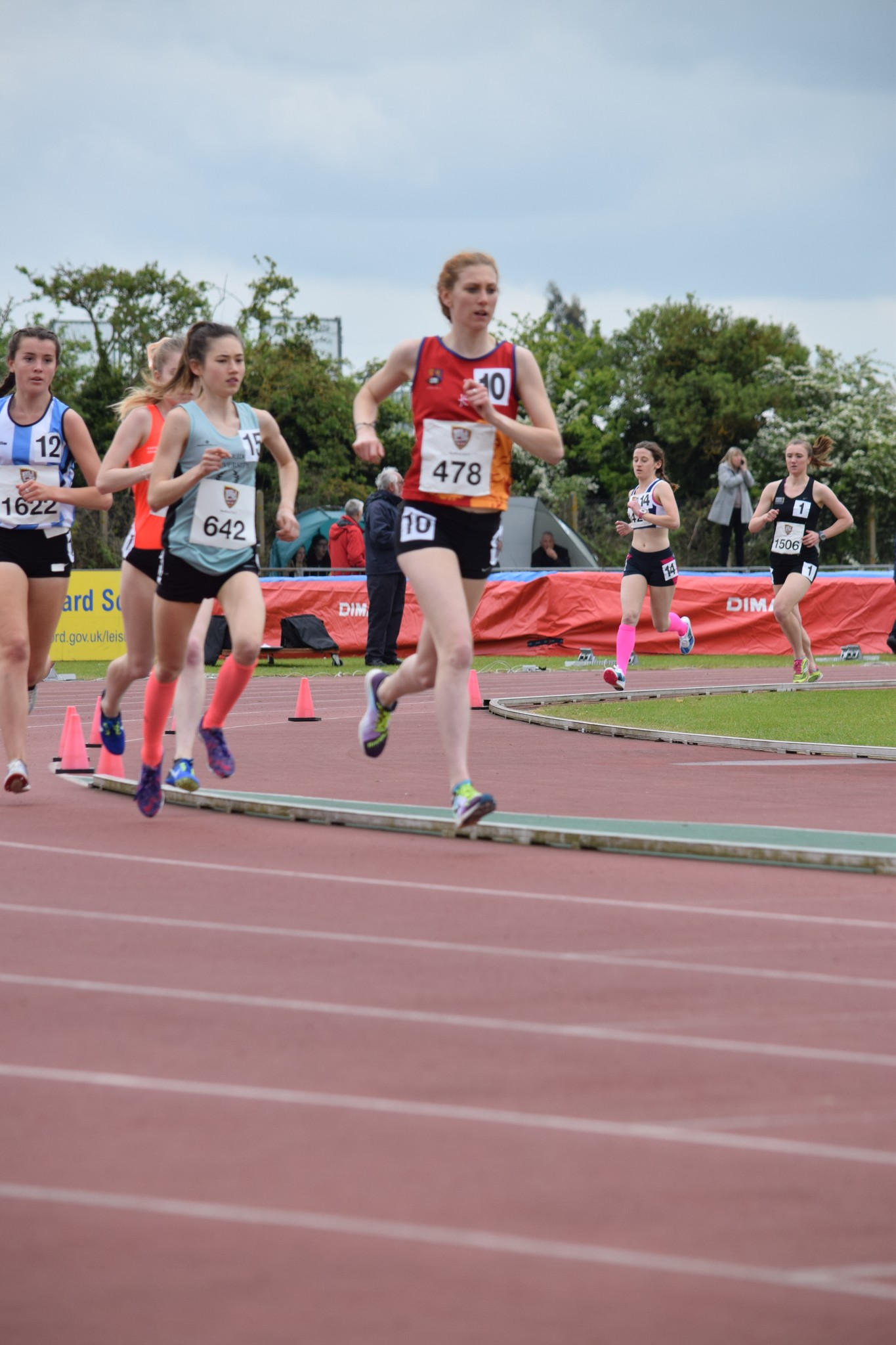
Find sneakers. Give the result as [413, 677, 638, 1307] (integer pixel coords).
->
[806, 665, 823, 683]
[135, 750, 165, 818]
[100, 688, 125, 755]
[677, 616, 694, 655]
[604, 664, 625, 691]
[791, 657, 810, 682]
[359, 668, 398, 758]
[197, 714, 236, 778]
[164, 758, 200, 792]
[452, 784, 496, 830]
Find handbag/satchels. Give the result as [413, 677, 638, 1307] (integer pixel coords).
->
[204, 615, 231, 665]
[280, 613, 338, 650]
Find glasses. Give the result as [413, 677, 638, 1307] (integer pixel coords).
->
[399, 480, 404, 484]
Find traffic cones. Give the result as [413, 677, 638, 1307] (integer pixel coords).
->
[287, 677, 322, 722]
[164, 713, 178, 734]
[467, 669, 489, 711]
[51, 706, 78, 762]
[82, 696, 105, 748]
[55, 714, 100, 774]
[94, 744, 126, 779]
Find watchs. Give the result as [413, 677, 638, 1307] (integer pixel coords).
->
[818, 530, 827, 541]
[639, 507, 646, 519]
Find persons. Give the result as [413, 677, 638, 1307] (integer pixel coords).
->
[491, 518, 504, 568]
[363, 466, 408, 666]
[603, 440, 695, 692]
[747, 433, 854, 683]
[329, 499, 367, 577]
[94, 335, 216, 791]
[0, 326, 114, 793]
[136, 320, 301, 818]
[708, 447, 756, 574]
[285, 545, 310, 577]
[305, 534, 332, 576]
[354, 249, 563, 830]
[531, 531, 571, 568]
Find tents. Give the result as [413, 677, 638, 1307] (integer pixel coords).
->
[268, 504, 365, 577]
[498, 495, 599, 568]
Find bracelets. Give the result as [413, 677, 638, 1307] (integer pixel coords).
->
[354, 420, 375, 426]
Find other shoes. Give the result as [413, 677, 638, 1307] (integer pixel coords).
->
[4, 758, 31, 793]
[365, 661, 384, 666]
[887, 637, 896, 654]
[386, 658, 402, 664]
[28, 685, 37, 713]
